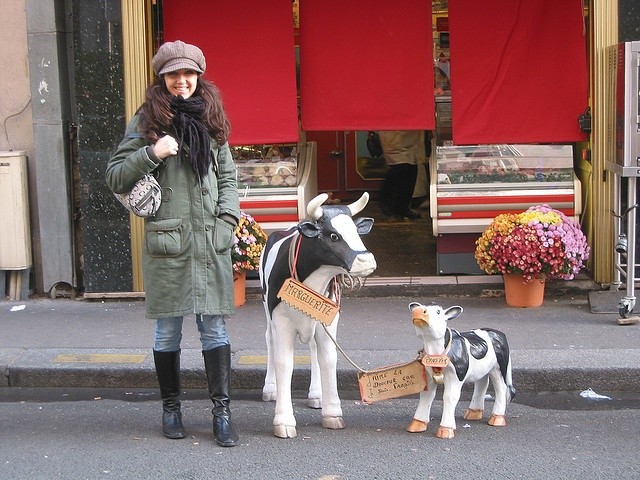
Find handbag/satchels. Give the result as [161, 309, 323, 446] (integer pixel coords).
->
[112, 174, 162, 219]
[366, 133, 382, 160]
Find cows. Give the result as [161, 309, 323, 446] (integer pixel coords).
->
[407, 301, 517, 438]
[259, 192, 378, 439]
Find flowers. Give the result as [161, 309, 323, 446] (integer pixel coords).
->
[475, 203, 590, 283]
[228, 210, 268, 281]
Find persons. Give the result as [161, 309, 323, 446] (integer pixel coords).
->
[376, 130, 422, 223]
[434, 66, 450, 90]
[104, 39, 242, 448]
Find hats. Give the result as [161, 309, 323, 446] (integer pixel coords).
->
[152, 40, 207, 78]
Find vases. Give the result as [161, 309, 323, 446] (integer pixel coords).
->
[234, 272, 246, 307]
[502, 272, 545, 307]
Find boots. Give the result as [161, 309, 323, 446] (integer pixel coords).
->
[202, 344, 240, 447]
[152, 348, 186, 439]
